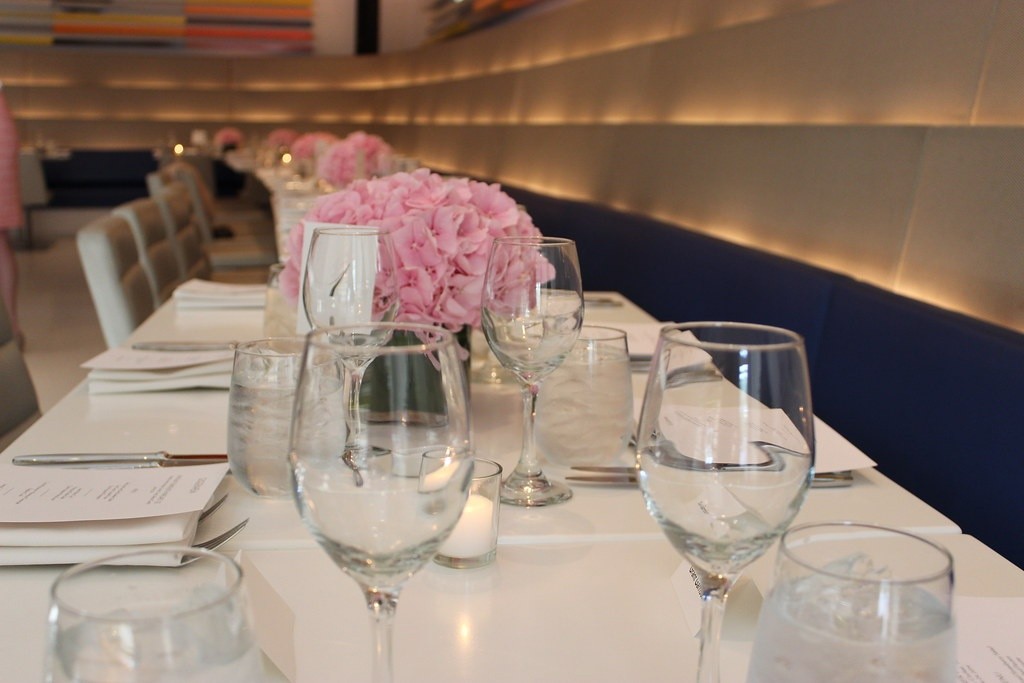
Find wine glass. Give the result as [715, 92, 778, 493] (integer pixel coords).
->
[482, 235, 585, 507]
[285, 327, 469, 683]
[303, 227, 398, 464]
[633, 322, 815, 683]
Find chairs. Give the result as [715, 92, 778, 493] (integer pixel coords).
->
[1, 123, 277, 463]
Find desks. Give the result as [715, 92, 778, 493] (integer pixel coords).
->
[8, 145, 51, 250]
[0, 276, 1024, 683]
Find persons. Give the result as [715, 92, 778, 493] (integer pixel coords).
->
[0, 92, 27, 349]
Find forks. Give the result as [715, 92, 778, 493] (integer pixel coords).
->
[197, 492, 228, 527]
[177, 519, 247, 567]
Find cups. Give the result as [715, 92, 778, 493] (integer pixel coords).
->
[538, 327, 634, 467]
[748, 521, 958, 683]
[228, 338, 307, 500]
[45, 546, 260, 683]
[434, 458, 503, 569]
[262, 264, 307, 336]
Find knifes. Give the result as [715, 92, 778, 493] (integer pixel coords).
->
[564, 466, 852, 488]
[12, 451, 226, 464]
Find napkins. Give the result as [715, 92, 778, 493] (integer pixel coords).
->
[86, 361, 231, 394]
[1, 492, 216, 567]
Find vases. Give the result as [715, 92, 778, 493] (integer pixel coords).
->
[331, 320, 472, 426]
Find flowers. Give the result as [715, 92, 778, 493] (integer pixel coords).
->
[214, 123, 556, 366]
